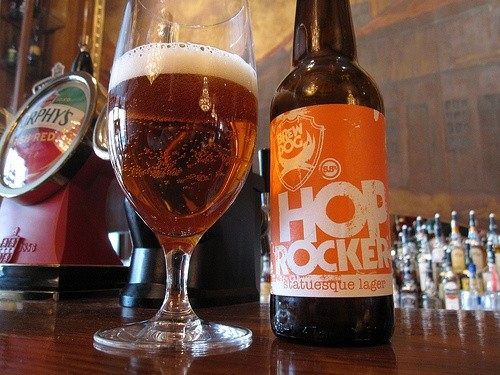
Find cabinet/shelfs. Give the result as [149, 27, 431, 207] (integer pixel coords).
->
[0, 4, 65, 84]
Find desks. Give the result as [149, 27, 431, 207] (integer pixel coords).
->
[0, 294, 500, 375]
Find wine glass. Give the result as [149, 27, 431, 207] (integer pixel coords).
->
[94, 0, 259, 351]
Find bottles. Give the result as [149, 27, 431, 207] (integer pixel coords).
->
[415, 212, 460, 310]
[9, 0, 40, 18]
[71, 35, 93, 76]
[27, 30, 41, 65]
[270, 0, 396, 346]
[392, 218, 419, 308]
[6, 35, 17, 67]
[449, 210, 487, 310]
[258, 192, 270, 303]
[483, 212, 500, 310]
